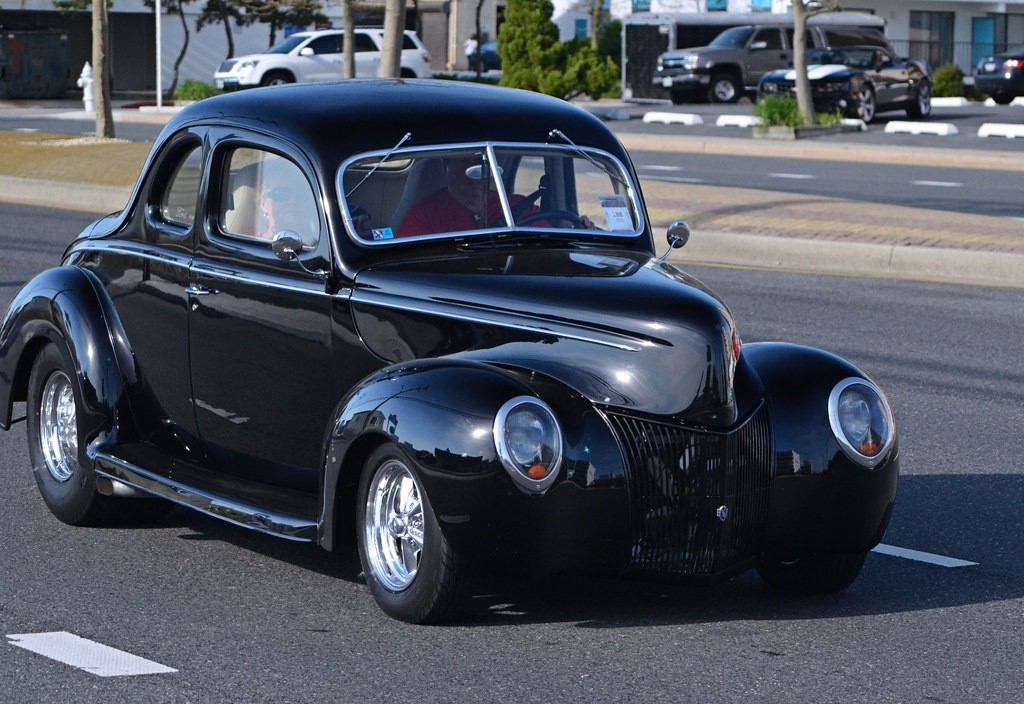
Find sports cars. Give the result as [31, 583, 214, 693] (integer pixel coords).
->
[0, 74, 904, 628]
[755, 44, 935, 124]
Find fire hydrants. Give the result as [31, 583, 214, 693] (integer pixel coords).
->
[76, 61, 95, 112]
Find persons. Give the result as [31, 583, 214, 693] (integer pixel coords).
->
[395, 150, 596, 237]
[463, 33, 479, 71]
[248, 162, 320, 250]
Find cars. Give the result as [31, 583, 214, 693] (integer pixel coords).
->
[469, 39, 502, 74]
[974, 45, 1024, 105]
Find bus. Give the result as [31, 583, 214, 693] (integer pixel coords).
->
[619, 7, 888, 106]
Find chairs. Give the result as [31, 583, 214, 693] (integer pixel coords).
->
[226, 157, 282, 239]
[387, 158, 449, 239]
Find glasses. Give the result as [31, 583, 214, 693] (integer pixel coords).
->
[263, 185, 312, 201]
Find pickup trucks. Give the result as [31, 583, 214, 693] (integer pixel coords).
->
[652, 19, 900, 105]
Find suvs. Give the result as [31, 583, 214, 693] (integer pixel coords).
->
[213, 25, 434, 92]
